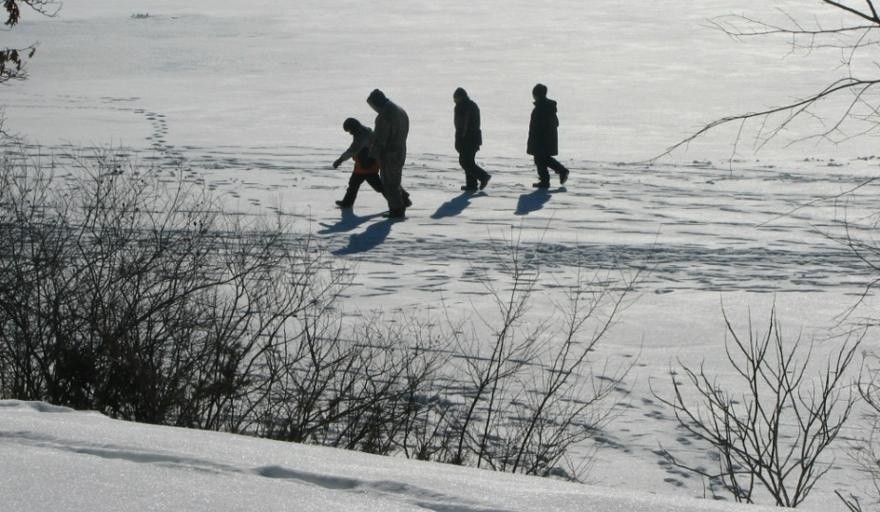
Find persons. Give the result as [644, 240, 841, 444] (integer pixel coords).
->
[333, 118, 386, 206]
[526, 84, 569, 188]
[367, 88, 413, 218]
[453, 88, 491, 190]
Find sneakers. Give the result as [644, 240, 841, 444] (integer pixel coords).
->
[383, 191, 412, 218]
[461, 184, 478, 190]
[336, 200, 353, 208]
[479, 174, 491, 190]
[560, 168, 569, 184]
[533, 181, 549, 188]
